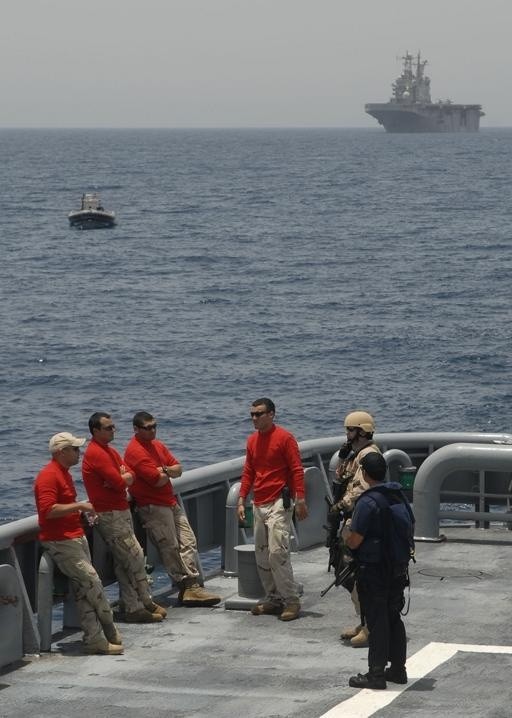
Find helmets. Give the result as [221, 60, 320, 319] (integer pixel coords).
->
[345, 411, 377, 439]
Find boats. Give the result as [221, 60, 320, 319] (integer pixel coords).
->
[68, 194, 117, 230]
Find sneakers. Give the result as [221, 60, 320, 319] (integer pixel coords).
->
[89, 588, 369, 659]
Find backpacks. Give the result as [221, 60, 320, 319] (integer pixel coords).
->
[363, 488, 416, 568]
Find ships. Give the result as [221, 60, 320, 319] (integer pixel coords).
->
[361, 48, 488, 134]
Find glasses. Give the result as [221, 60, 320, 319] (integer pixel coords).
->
[249, 409, 274, 417]
[104, 423, 158, 432]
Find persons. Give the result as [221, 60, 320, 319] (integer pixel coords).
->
[237, 398, 309, 620]
[342, 451, 416, 690]
[82, 412, 167, 622]
[34, 431, 124, 655]
[125, 412, 221, 606]
[328, 411, 383, 647]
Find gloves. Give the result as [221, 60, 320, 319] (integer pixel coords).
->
[330, 502, 346, 512]
[338, 442, 352, 459]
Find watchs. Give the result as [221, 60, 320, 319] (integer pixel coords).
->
[162, 465, 168, 473]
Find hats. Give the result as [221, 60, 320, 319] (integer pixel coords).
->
[49, 431, 86, 453]
[358, 452, 386, 481]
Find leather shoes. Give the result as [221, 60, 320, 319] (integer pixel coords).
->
[349, 663, 410, 688]
